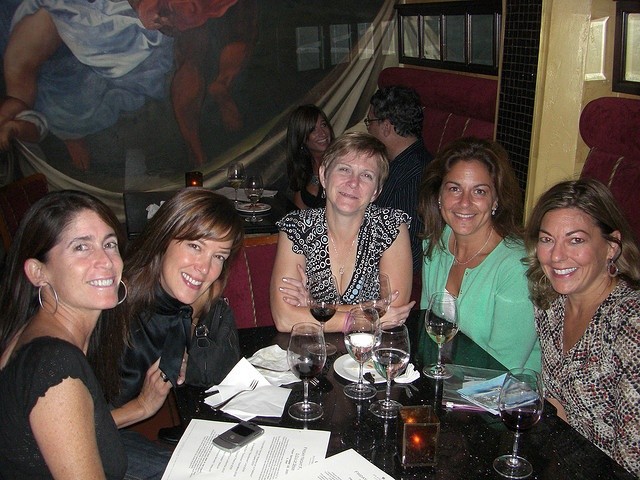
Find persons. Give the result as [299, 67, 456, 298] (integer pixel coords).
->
[108, 187, 244, 441]
[525, 178, 639, 480]
[0, 188, 131, 476]
[358, 82, 440, 269]
[419, 137, 545, 379]
[287, 97, 333, 209]
[270, 130, 415, 338]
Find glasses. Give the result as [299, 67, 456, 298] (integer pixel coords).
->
[364, 117, 384, 126]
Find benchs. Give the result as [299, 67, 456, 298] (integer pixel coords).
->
[220, 234, 281, 330]
[377, 67, 499, 159]
[0, 174, 49, 254]
[574, 98, 640, 250]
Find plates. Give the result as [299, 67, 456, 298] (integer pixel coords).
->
[333, 354, 387, 384]
[235, 203, 271, 214]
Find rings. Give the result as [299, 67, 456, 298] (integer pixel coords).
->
[159, 372, 167, 381]
[296, 302, 302, 307]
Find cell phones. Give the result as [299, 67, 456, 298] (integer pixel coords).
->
[212, 420, 265, 455]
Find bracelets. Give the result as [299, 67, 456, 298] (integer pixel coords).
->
[341, 310, 354, 334]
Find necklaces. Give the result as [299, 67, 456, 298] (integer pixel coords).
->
[309, 172, 320, 185]
[451, 228, 492, 266]
[323, 219, 363, 275]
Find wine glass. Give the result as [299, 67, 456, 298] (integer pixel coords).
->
[369, 320, 411, 419]
[372, 422, 395, 474]
[342, 402, 375, 451]
[358, 273, 391, 332]
[244, 175, 263, 222]
[308, 275, 338, 357]
[422, 292, 460, 379]
[343, 307, 378, 400]
[493, 368, 544, 479]
[287, 322, 326, 422]
[227, 161, 247, 205]
